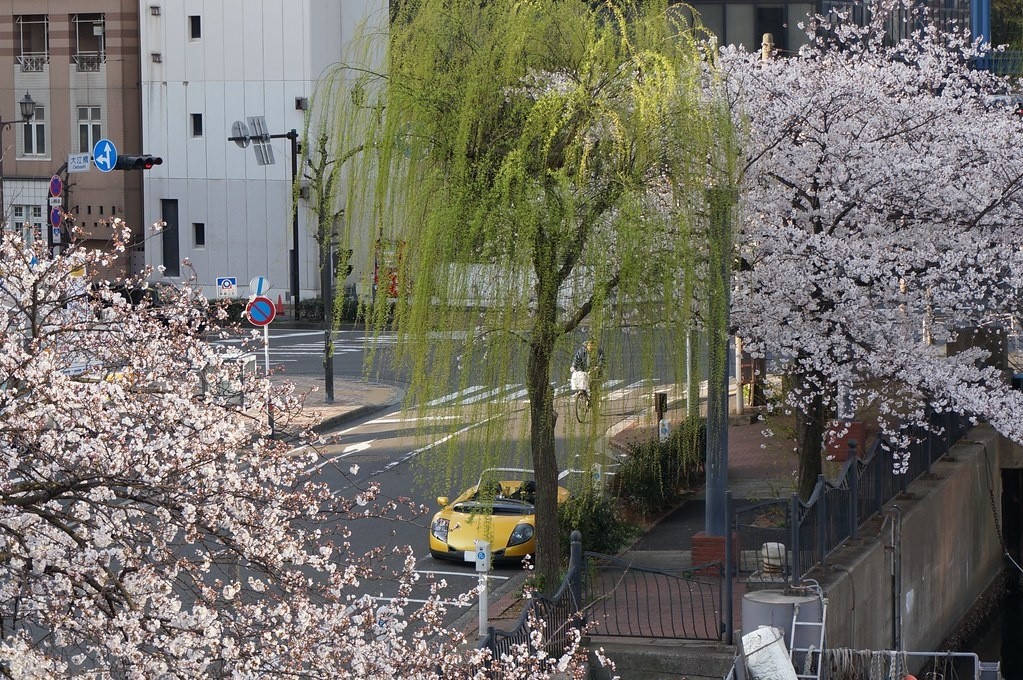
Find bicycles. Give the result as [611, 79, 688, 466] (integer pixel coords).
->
[570, 368, 604, 422]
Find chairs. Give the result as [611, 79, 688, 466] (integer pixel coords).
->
[474, 481, 506, 501]
[509, 481, 535, 505]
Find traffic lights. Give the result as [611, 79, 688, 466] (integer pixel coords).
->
[122, 152, 163, 170]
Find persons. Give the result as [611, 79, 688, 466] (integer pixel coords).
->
[896, 277, 937, 348]
[570, 338, 606, 415]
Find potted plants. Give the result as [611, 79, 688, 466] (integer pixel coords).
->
[559, 418, 707, 573]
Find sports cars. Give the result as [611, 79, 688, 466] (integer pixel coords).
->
[428, 479, 573, 564]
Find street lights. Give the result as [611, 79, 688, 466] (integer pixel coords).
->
[0, 88, 37, 262]
